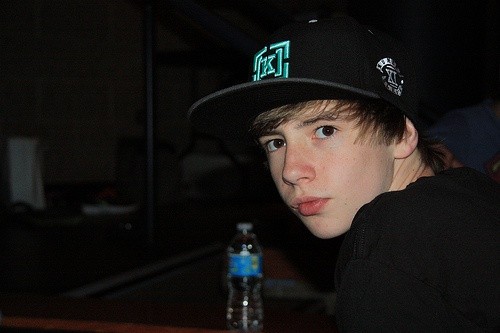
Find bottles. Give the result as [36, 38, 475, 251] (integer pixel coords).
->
[226, 222, 264, 333]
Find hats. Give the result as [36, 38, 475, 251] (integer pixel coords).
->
[188, 15, 418, 137]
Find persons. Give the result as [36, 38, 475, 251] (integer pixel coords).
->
[423, 95, 500, 187]
[187, 17, 500, 333]
